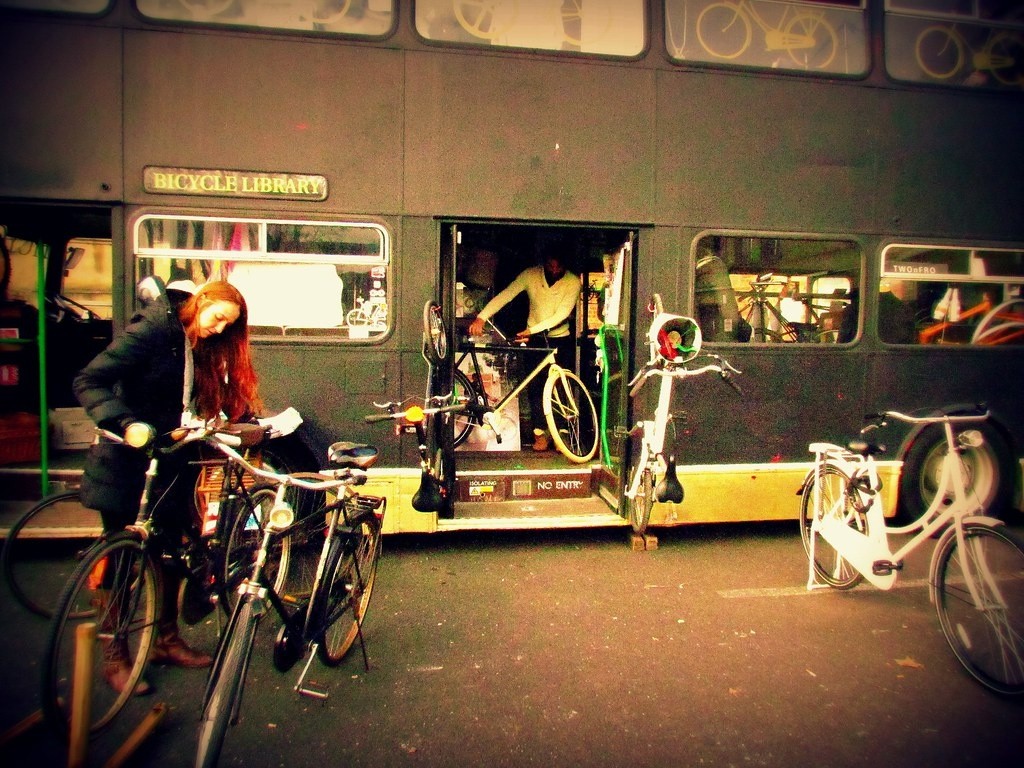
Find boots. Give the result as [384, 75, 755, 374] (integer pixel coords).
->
[147, 560, 213, 668]
[96, 589, 149, 695]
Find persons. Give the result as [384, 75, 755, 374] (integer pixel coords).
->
[72, 281, 262, 696]
[468, 241, 581, 451]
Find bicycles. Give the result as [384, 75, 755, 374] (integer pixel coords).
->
[745, 268, 852, 345]
[0, 406, 297, 739]
[796, 411, 1024, 700]
[446, 322, 601, 464]
[180, 428, 387, 767]
[364, 298, 494, 515]
[623, 294, 746, 539]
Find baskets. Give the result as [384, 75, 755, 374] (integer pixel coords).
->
[650, 313, 702, 363]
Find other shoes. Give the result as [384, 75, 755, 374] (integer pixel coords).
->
[556, 429, 570, 452]
[533, 428, 548, 450]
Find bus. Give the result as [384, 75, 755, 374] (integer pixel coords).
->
[0, 1, 1023, 542]
